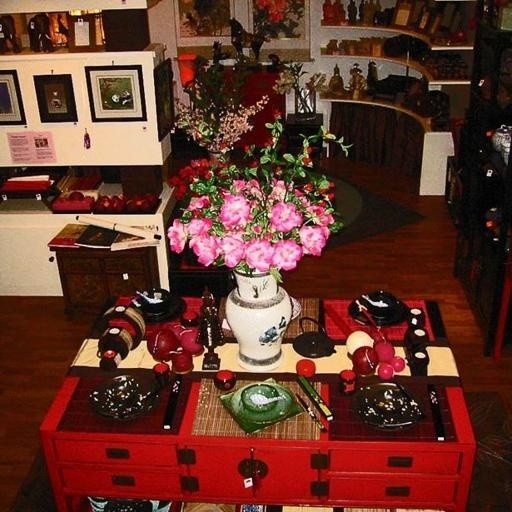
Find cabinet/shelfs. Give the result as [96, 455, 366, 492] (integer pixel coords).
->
[0, 0, 179, 300]
[36, 292, 475, 512]
[318, 18, 477, 197]
[51, 242, 159, 316]
[284, 111, 323, 156]
[454, 0, 512, 360]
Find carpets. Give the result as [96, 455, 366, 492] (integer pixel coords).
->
[281, 145, 430, 257]
[10, 385, 511, 512]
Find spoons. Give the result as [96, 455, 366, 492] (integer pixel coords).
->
[133, 288, 163, 305]
[361, 293, 389, 310]
[249, 393, 286, 405]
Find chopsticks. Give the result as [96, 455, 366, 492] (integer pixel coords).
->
[294, 391, 328, 437]
[393, 374, 422, 418]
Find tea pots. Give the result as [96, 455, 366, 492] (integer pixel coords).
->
[293, 315, 339, 358]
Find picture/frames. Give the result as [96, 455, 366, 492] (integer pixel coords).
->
[0, 68, 28, 127]
[84, 65, 148, 125]
[32, 72, 80, 125]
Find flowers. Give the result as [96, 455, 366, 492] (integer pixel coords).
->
[272, 59, 330, 112]
[160, 117, 355, 283]
[167, 57, 271, 158]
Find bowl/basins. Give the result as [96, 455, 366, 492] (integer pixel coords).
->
[87, 372, 161, 420]
[350, 380, 423, 429]
[360, 288, 401, 321]
[137, 287, 176, 316]
[240, 384, 279, 412]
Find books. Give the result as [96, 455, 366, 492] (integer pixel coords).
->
[110, 224, 161, 252]
[74, 224, 120, 249]
[47, 224, 90, 249]
[67, 171, 104, 192]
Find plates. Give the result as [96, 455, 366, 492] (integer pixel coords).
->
[219, 376, 304, 437]
[229, 381, 295, 424]
[347, 299, 410, 329]
[128, 294, 187, 324]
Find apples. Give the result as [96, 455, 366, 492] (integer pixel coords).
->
[352, 346, 378, 374]
[147, 328, 178, 362]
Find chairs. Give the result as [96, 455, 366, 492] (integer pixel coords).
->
[202, 62, 286, 156]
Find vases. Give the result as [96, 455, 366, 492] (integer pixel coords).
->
[225, 261, 294, 372]
